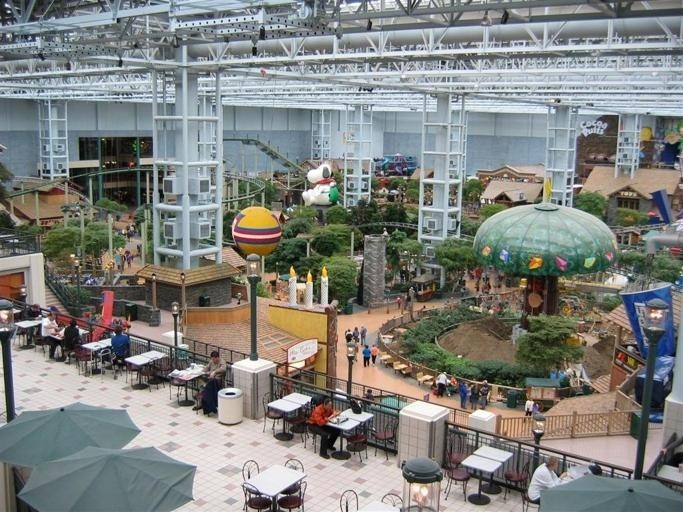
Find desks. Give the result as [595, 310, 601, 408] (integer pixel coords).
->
[472, 446, 513, 495]
[461, 454, 501, 505]
[359, 500, 399, 511]
[241, 464, 308, 512]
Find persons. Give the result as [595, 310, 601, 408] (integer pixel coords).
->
[40, 313, 64, 361]
[197, 350, 226, 386]
[522, 397, 534, 422]
[435, 370, 447, 397]
[307, 397, 339, 460]
[478, 379, 489, 409]
[191, 375, 214, 413]
[111, 325, 130, 367]
[397, 293, 411, 311]
[445, 375, 457, 397]
[527, 455, 567, 507]
[458, 381, 467, 409]
[62, 319, 80, 351]
[459, 271, 505, 298]
[19, 304, 41, 347]
[469, 384, 477, 409]
[344, 323, 378, 367]
[105, 210, 142, 268]
[363, 388, 375, 407]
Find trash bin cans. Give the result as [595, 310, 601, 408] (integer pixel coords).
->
[345, 303, 353, 315]
[125, 303, 137, 321]
[507, 389, 517, 408]
[630, 412, 649, 440]
[217, 387, 244, 425]
[199, 296, 210, 307]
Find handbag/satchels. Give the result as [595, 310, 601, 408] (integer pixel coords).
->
[350, 399, 361, 414]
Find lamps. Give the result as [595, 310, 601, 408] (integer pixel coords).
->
[25, 16, 274, 77]
[498, 7, 511, 25]
[363, 17, 375, 32]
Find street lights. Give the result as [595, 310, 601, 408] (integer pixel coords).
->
[69, 254, 74, 285]
[179, 272, 185, 315]
[399, 457, 443, 512]
[171, 301, 178, 369]
[0, 299, 15, 424]
[633, 297, 668, 480]
[346, 340, 356, 400]
[73, 257, 80, 304]
[18, 284, 26, 304]
[150, 273, 156, 310]
[246, 254, 261, 361]
[531, 414, 546, 478]
[107, 260, 114, 286]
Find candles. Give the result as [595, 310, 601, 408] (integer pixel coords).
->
[287, 266, 298, 303]
[304, 269, 313, 306]
[320, 267, 330, 303]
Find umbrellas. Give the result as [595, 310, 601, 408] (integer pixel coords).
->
[538, 472, 683, 511]
[0, 400, 141, 469]
[11, 441, 197, 512]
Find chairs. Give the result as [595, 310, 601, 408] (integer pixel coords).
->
[339, 489, 359, 511]
[440, 429, 470, 502]
[242, 459, 260, 481]
[503, 459, 540, 511]
[277, 481, 308, 512]
[379, 349, 526, 407]
[374, 418, 399, 460]
[241, 483, 273, 512]
[0, 304, 226, 418]
[284, 458, 305, 474]
[381, 492, 403, 507]
[261, 387, 374, 463]
